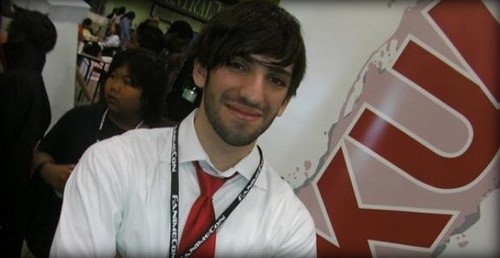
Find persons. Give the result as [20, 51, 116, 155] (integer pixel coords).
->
[79, 3, 205, 119]
[48, 0, 317, 258]
[0, 2, 58, 258]
[20, 48, 166, 258]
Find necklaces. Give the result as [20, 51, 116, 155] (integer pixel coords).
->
[94, 108, 144, 144]
[169, 122, 264, 258]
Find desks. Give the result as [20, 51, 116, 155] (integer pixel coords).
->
[77, 41, 112, 105]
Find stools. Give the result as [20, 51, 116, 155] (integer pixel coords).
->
[85, 67, 107, 92]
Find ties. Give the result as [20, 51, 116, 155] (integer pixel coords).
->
[177, 160, 241, 258]
